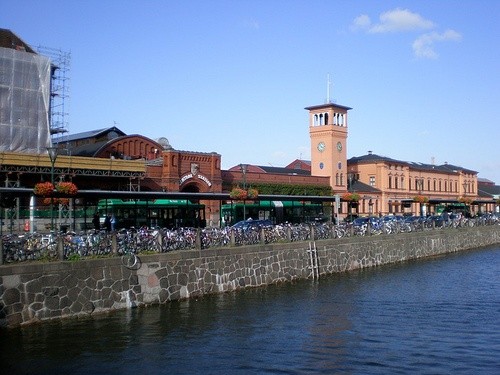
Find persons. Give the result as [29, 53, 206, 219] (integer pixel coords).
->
[93, 212, 125, 232]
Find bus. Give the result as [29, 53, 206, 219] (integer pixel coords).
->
[435, 203, 470, 218]
[222, 199, 325, 224]
[98, 198, 197, 230]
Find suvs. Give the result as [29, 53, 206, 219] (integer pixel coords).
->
[233, 219, 273, 229]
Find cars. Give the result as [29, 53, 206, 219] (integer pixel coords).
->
[350, 216, 380, 226]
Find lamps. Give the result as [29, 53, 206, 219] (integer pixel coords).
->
[317, 142, 325, 152]
[337, 142, 342, 151]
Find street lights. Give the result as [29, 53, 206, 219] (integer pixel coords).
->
[46, 146, 61, 232]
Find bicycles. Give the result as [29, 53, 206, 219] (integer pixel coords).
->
[236, 221, 377, 246]
[376, 210, 500, 234]
[0, 225, 237, 264]
[122, 235, 139, 269]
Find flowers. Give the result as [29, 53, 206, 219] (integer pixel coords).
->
[459, 198, 473, 203]
[230, 188, 258, 200]
[342, 193, 361, 201]
[413, 196, 429, 203]
[34, 181, 77, 197]
